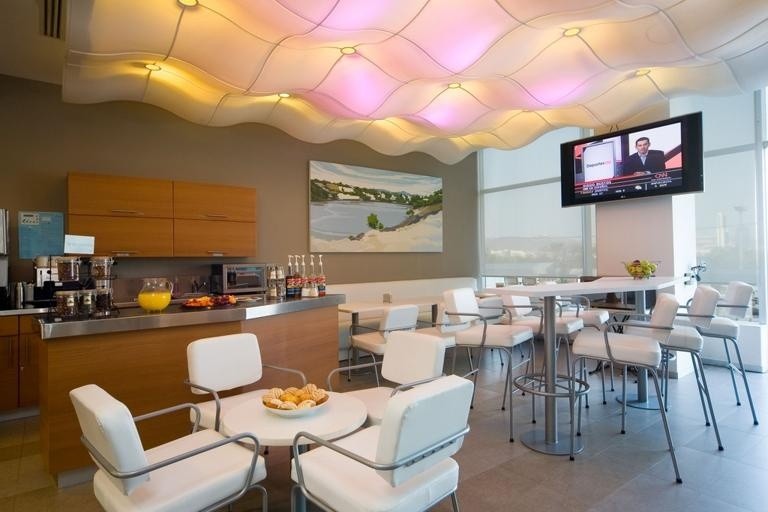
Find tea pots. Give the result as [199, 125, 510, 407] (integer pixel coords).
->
[137, 278, 173, 312]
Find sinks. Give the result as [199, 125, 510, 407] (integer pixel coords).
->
[184, 293, 209, 296]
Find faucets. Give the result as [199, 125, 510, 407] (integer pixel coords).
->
[193, 280, 207, 292]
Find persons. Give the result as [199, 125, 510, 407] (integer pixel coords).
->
[623, 137, 665, 176]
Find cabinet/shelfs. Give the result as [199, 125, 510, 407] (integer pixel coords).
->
[64, 172, 258, 257]
[1, 314, 39, 412]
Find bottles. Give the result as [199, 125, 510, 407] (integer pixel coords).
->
[265, 264, 287, 296]
[10, 282, 35, 309]
[173, 279, 180, 299]
[55, 256, 113, 321]
[302, 283, 311, 297]
[310, 283, 319, 297]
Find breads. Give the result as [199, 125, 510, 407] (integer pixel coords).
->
[262, 383, 329, 410]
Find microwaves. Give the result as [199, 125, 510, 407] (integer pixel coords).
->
[209, 263, 267, 294]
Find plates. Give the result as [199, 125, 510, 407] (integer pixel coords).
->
[260, 394, 331, 418]
[181, 303, 211, 309]
[235, 297, 263, 302]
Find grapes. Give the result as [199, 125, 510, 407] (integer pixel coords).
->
[210, 295, 228, 305]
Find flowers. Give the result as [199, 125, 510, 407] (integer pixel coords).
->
[622, 260, 658, 277]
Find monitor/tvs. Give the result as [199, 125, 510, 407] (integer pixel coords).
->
[560, 111, 704, 206]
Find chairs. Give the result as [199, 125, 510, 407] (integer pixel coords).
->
[569, 293, 682, 483]
[290, 374, 474, 512]
[664, 280, 760, 425]
[622, 286, 724, 450]
[187, 333, 307, 444]
[70, 383, 268, 511]
